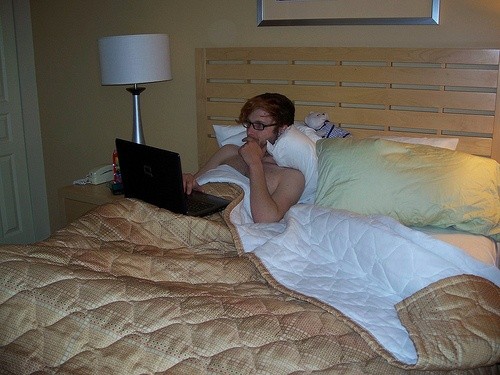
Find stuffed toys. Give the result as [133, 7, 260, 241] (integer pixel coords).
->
[304, 110, 353, 139]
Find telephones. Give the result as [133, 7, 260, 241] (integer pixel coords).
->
[72, 164, 114, 185]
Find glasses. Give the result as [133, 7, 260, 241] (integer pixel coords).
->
[241, 120, 279, 130]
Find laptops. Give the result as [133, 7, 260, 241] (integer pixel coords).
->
[115, 137, 232, 217]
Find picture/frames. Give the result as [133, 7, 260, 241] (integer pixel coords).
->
[255, 0, 439, 26]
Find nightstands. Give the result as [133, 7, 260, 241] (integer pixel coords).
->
[65, 180, 124, 221]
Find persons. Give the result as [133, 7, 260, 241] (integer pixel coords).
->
[182, 92, 306, 223]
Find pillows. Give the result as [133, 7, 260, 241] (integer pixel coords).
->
[212, 123, 500, 242]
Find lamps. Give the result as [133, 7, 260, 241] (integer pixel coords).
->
[97, 34, 173, 145]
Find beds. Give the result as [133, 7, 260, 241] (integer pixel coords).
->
[0, 47, 500, 375]
